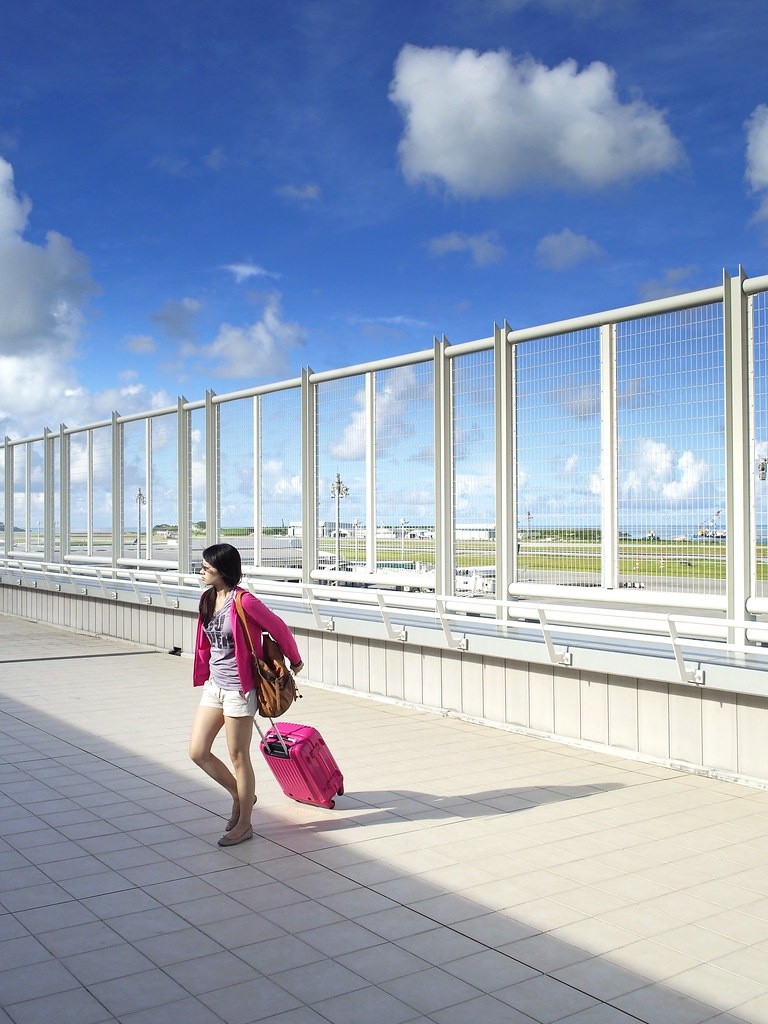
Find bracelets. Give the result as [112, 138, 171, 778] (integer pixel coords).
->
[290, 660, 302, 667]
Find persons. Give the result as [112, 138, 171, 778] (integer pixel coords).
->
[188, 543, 304, 847]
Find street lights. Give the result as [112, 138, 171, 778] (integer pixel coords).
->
[135, 487, 147, 571]
[351, 518, 363, 562]
[328, 473, 350, 587]
[399, 517, 410, 561]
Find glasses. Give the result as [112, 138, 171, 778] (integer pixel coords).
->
[199, 561, 220, 576]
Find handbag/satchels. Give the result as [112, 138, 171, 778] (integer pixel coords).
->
[235, 590, 303, 718]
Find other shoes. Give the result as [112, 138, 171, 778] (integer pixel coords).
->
[217, 823, 253, 847]
[224, 795, 258, 832]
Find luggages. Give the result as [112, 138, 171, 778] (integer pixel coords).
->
[252, 716, 344, 809]
